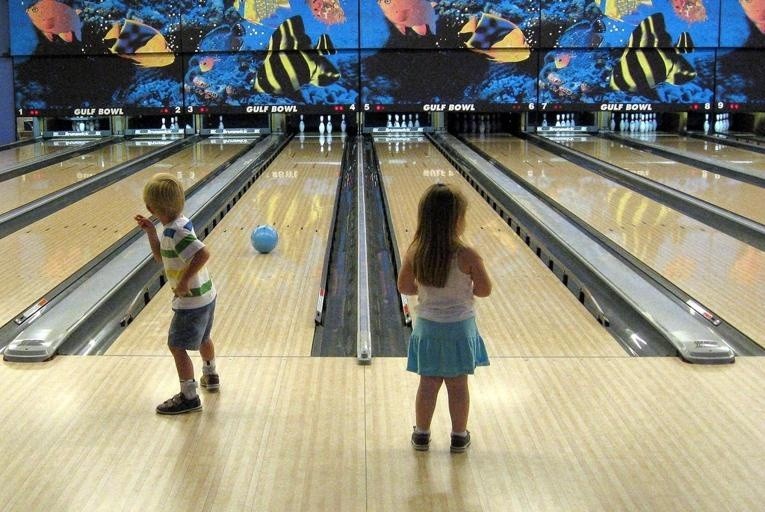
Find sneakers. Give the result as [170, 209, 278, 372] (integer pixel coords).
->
[411, 425, 432, 452]
[200, 373, 220, 391]
[155, 391, 203, 414]
[449, 430, 472, 452]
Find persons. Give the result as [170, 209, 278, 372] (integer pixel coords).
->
[134, 172, 221, 417]
[394, 182, 494, 455]
